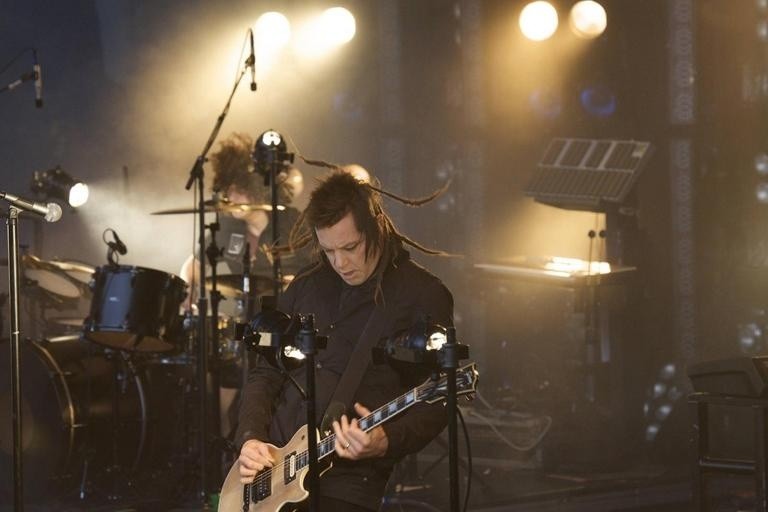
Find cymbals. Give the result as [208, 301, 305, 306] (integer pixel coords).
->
[205, 273, 288, 297]
[148, 202, 289, 215]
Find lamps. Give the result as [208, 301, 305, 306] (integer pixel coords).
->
[24, 165, 91, 210]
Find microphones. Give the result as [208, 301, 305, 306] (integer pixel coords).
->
[31, 47, 45, 111]
[248, 29, 259, 94]
[0, 189, 64, 225]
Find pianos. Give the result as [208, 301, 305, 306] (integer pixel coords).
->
[522, 138, 655, 204]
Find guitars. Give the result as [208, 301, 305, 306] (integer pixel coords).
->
[215, 360, 478, 511]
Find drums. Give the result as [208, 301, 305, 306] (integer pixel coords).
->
[46, 261, 95, 290]
[47, 316, 88, 339]
[21, 269, 80, 312]
[165, 308, 235, 357]
[1, 333, 154, 512]
[80, 263, 189, 357]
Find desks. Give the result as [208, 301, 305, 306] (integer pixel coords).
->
[688, 392, 768, 511]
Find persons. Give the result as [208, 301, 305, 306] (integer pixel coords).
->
[174, 133, 317, 448]
[229, 169, 460, 511]
